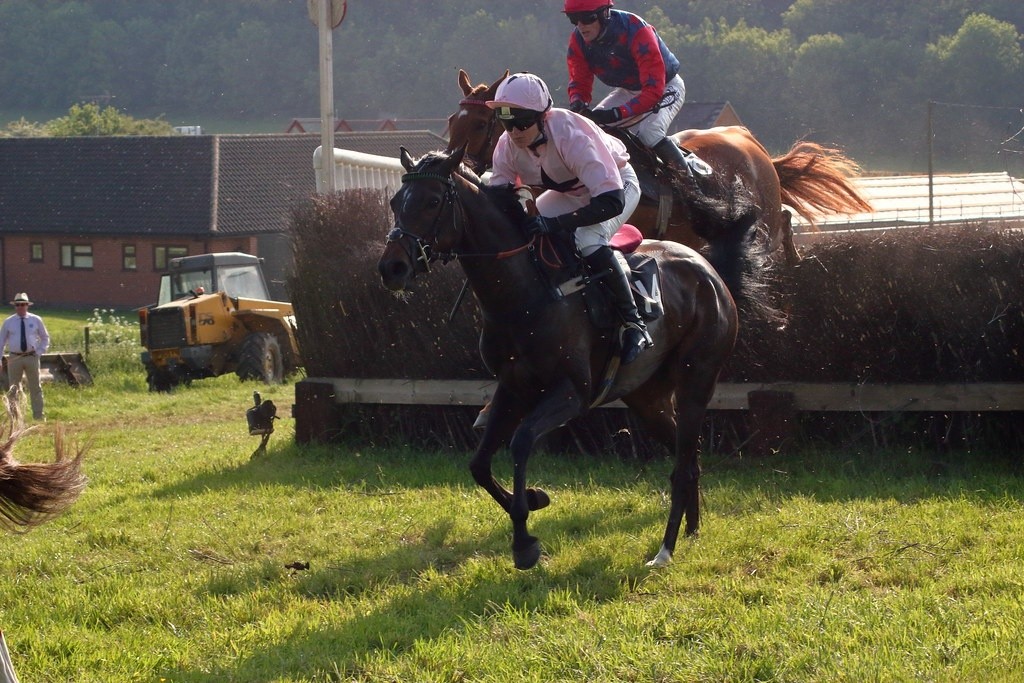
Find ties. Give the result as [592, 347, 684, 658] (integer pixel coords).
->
[20, 317, 27, 352]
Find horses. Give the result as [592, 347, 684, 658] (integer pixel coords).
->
[446, 70, 873, 460]
[378, 146, 788, 571]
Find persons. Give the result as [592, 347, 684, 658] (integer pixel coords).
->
[480, 70, 654, 365]
[0, 292, 50, 422]
[561, 0, 703, 196]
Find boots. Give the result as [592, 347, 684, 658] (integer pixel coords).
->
[652, 137, 703, 206]
[582, 245, 654, 364]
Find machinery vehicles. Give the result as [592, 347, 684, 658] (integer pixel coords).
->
[139, 250, 303, 395]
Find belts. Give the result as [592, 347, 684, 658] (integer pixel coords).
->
[11, 352, 34, 356]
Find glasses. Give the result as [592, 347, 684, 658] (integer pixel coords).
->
[14, 303, 25, 306]
[500, 119, 537, 132]
[570, 12, 599, 25]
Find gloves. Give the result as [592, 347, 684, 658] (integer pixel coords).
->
[522, 215, 561, 236]
[569, 100, 622, 127]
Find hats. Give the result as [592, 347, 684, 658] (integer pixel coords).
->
[485, 72, 554, 112]
[10, 293, 34, 305]
[560, 0, 614, 13]
